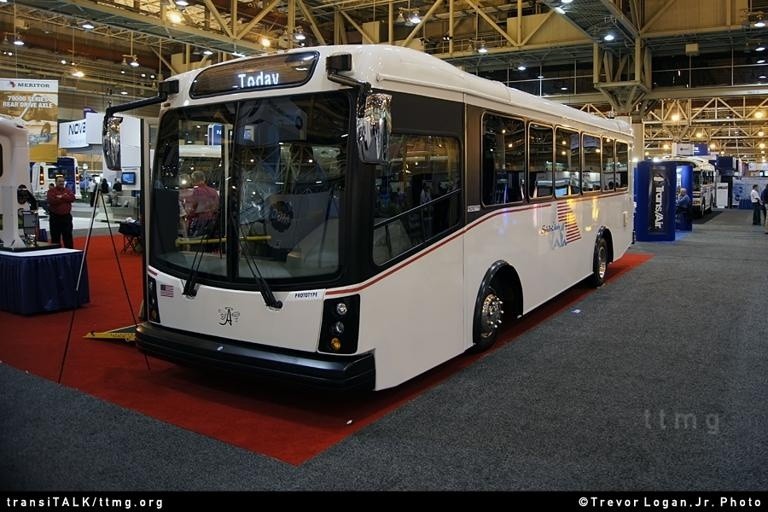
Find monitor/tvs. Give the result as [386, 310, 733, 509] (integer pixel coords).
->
[23, 213, 39, 228]
[121, 172, 135, 184]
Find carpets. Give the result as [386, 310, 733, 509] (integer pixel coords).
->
[0, 233, 653, 466]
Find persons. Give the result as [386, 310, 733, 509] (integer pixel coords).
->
[675, 188, 690, 214]
[289, 140, 328, 193]
[186, 170, 220, 254]
[49, 183, 54, 189]
[47, 176, 75, 249]
[90, 178, 122, 207]
[751, 184, 762, 225]
[238, 138, 280, 256]
[761, 184, 768, 226]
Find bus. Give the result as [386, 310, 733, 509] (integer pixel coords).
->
[102, 44, 634, 395]
[653, 155, 717, 217]
[30, 155, 82, 213]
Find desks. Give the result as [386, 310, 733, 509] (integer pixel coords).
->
[118, 221, 141, 255]
[0, 240, 90, 316]
